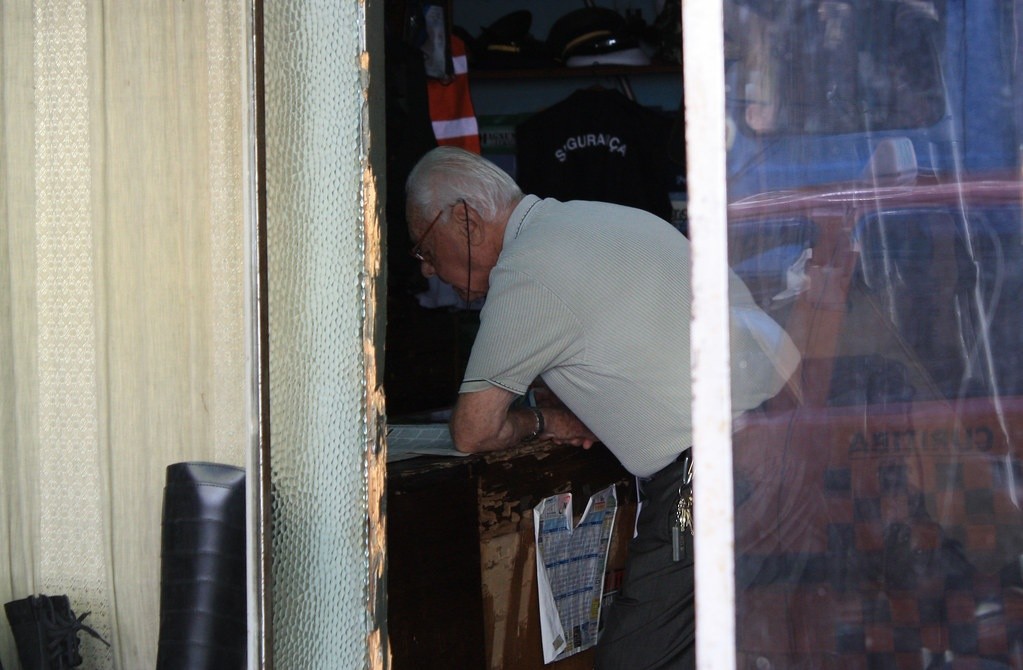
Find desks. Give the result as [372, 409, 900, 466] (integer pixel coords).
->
[388, 404, 642, 670]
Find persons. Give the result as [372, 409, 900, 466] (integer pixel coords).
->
[405, 146, 828, 668]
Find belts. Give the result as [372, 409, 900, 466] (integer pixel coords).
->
[671, 397, 793, 464]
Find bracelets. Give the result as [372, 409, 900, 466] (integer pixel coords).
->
[522, 401, 546, 441]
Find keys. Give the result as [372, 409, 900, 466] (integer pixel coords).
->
[674, 486, 695, 534]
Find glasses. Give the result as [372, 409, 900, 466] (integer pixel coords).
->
[409, 210, 443, 261]
